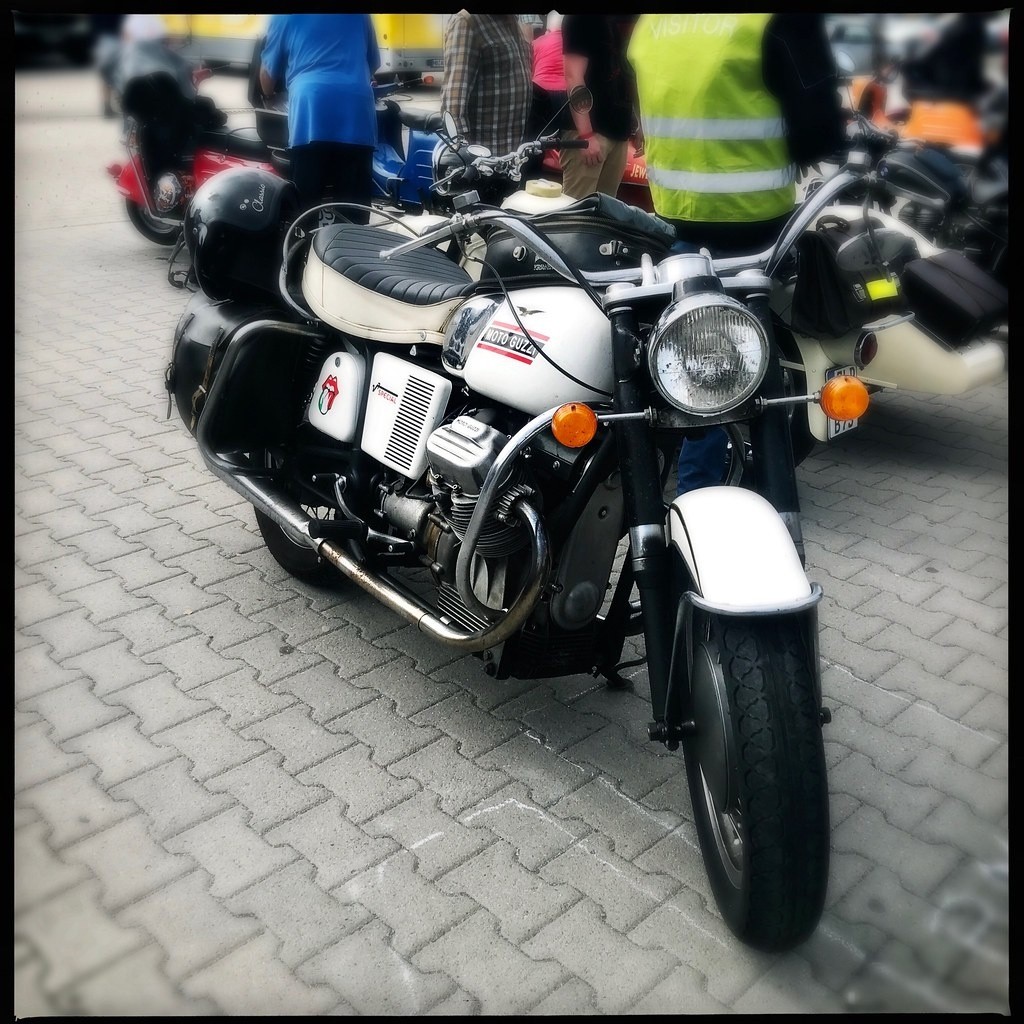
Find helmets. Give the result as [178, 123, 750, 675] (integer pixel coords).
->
[183, 167, 303, 301]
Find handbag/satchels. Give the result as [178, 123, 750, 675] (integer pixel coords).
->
[164, 292, 301, 442]
[793, 214, 887, 342]
[479, 195, 677, 283]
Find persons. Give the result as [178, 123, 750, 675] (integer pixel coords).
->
[623, 8, 852, 512]
[85, 6, 644, 210]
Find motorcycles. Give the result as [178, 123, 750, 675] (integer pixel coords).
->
[91, 8, 1013, 951]
